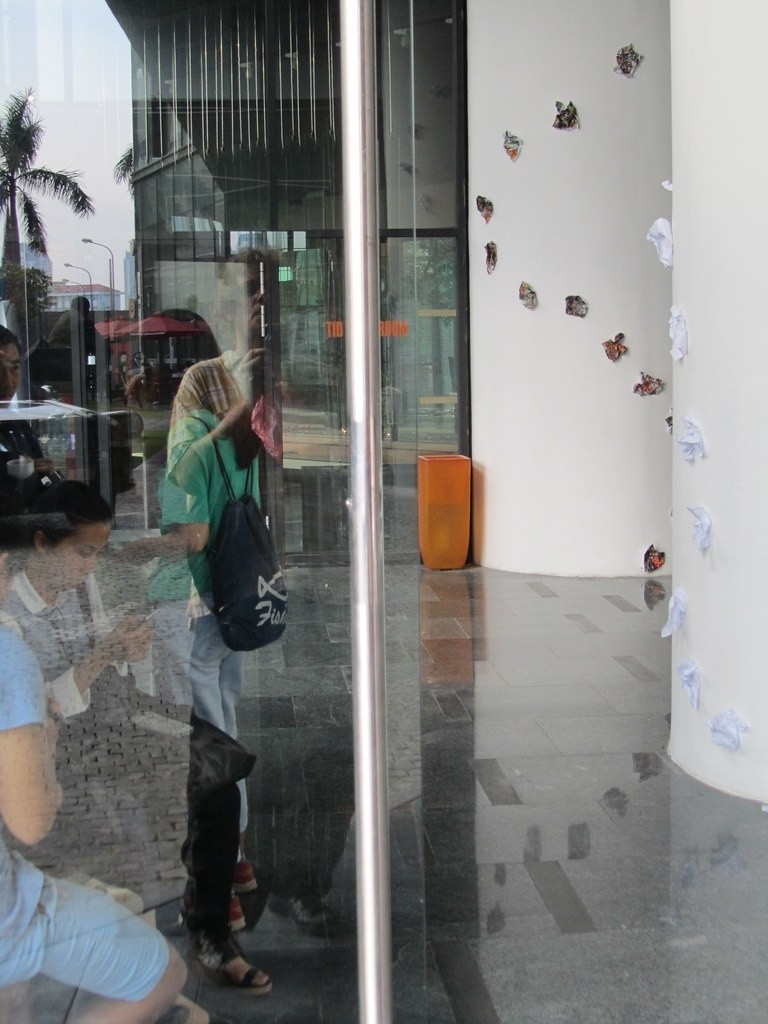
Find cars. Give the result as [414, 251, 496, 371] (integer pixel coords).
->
[0, 298, 144, 551]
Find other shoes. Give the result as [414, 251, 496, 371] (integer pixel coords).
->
[196, 936, 272, 995]
[229, 898, 246, 932]
[233, 860, 259, 894]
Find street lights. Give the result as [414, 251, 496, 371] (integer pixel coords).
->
[82, 235, 117, 323]
[63, 262, 94, 312]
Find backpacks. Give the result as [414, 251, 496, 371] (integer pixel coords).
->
[190, 413, 288, 650]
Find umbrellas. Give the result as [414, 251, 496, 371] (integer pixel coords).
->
[91, 311, 211, 337]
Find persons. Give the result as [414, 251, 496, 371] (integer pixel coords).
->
[0, 288, 386, 1024]
[164, 244, 399, 996]
